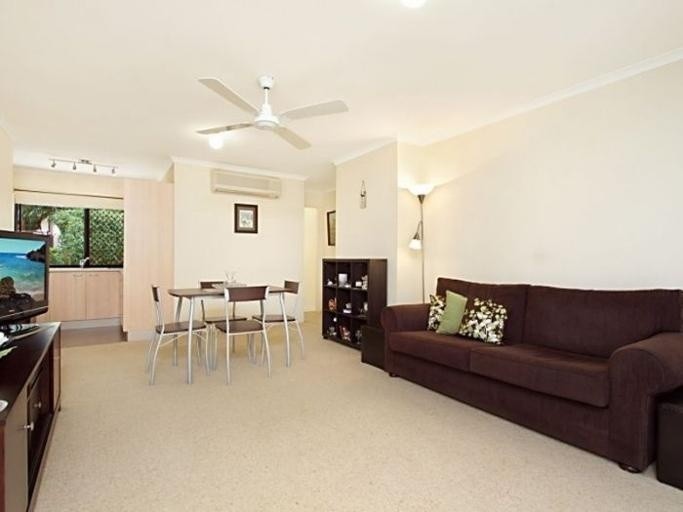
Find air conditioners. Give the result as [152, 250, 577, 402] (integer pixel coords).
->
[211, 171, 284, 199]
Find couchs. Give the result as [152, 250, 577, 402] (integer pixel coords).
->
[383, 277, 683, 473]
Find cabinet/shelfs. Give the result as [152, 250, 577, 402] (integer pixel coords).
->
[322, 257, 387, 349]
[0, 321, 62, 511]
[361, 325, 384, 369]
[49, 272, 120, 320]
[658, 398, 683, 491]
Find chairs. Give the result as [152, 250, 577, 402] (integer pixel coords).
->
[213, 286, 272, 383]
[197, 280, 247, 366]
[148, 283, 211, 383]
[250, 280, 306, 377]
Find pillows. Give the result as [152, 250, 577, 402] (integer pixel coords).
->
[459, 295, 513, 345]
[426, 294, 469, 331]
[436, 289, 468, 335]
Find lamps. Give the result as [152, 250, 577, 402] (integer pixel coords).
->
[359, 179, 367, 210]
[48, 159, 122, 177]
[408, 183, 434, 304]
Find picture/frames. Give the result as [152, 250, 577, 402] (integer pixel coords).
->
[233, 204, 258, 234]
[326, 210, 336, 247]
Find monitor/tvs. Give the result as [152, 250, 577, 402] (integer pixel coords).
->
[0, 230, 49, 336]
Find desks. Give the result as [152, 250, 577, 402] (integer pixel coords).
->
[167, 287, 293, 386]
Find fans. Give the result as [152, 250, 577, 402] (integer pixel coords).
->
[196, 74, 349, 152]
